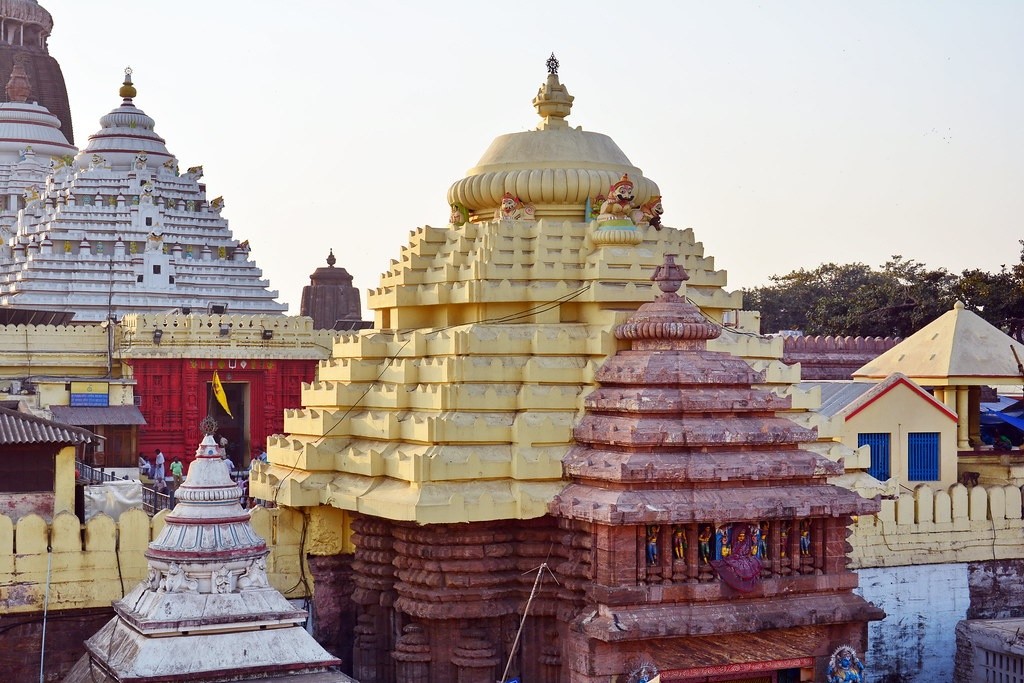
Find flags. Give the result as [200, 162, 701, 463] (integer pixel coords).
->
[213, 369, 233, 419]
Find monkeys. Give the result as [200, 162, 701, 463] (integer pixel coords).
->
[959, 469, 981, 487]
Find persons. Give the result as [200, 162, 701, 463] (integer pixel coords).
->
[671, 522, 688, 560]
[711, 525, 763, 592]
[698, 525, 712, 566]
[780, 520, 791, 559]
[249, 450, 268, 470]
[826, 644, 864, 683]
[800, 517, 813, 558]
[154, 449, 165, 481]
[169, 456, 184, 487]
[760, 519, 770, 561]
[137, 451, 154, 479]
[222, 454, 235, 479]
[151, 476, 168, 495]
[646, 524, 660, 567]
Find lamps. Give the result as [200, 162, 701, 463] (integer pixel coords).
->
[152, 329, 164, 347]
[262, 329, 273, 340]
